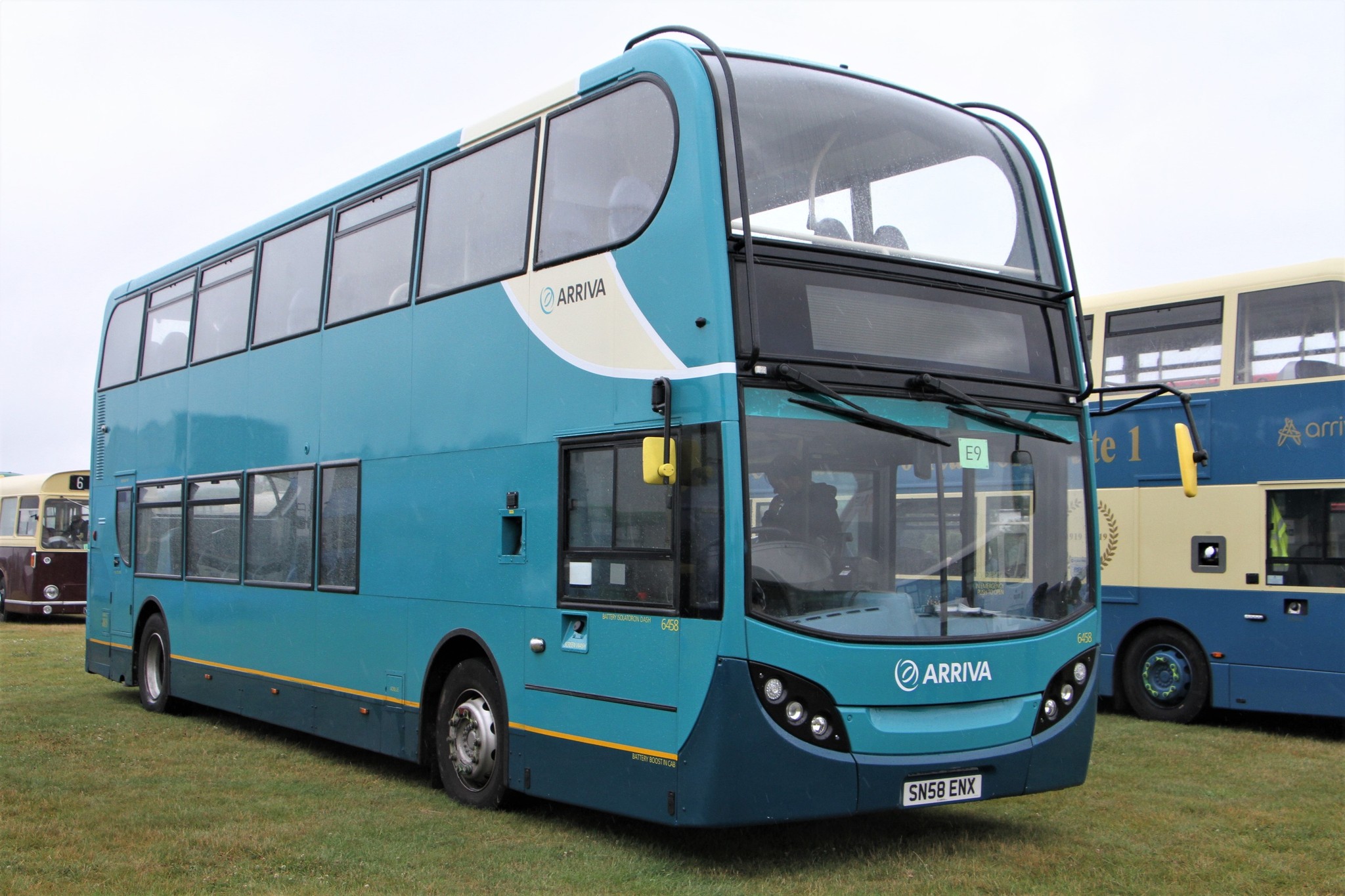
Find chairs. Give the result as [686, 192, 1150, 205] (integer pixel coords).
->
[1280, 359, 1342, 380]
[135, 512, 239, 582]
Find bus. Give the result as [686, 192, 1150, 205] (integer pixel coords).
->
[750, 261, 1345, 724]
[85, 23, 1208, 833]
[0, 469, 94, 618]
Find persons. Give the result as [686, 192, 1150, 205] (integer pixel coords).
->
[759, 453, 855, 594]
[60, 515, 88, 541]
